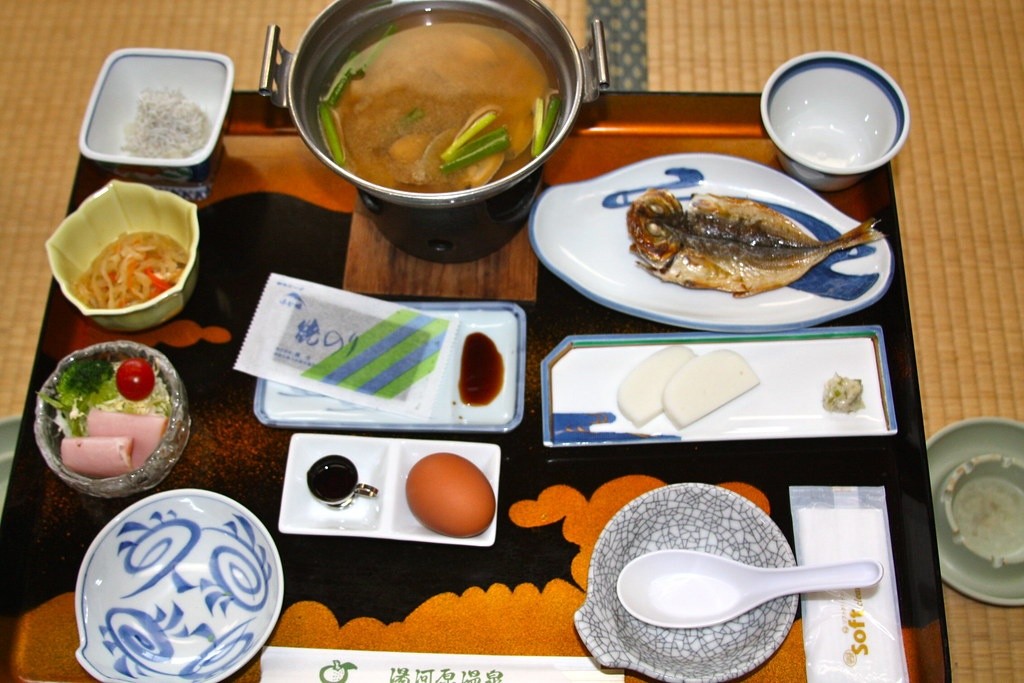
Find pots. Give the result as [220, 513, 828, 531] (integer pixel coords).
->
[258, 0, 613, 207]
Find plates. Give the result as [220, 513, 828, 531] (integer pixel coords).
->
[541, 325, 897, 446]
[278, 432, 500, 547]
[526, 153, 895, 333]
[253, 301, 527, 433]
[927, 416, 1024, 607]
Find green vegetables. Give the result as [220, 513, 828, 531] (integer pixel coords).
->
[316, 23, 562, 175]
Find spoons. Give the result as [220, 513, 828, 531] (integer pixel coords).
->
[616, 550, 885, 628]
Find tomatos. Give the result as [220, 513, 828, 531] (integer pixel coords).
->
[116, 358, 156, 402]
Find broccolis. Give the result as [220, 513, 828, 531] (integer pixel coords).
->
[36, 358, 118, 437]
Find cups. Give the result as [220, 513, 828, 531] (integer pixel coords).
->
[305, 455, 379, 509]
[759, 50, 910, 193]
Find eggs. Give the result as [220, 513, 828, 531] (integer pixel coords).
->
[405, 453, 495, 538]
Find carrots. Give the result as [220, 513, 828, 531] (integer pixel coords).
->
[106, 259, 173, 301]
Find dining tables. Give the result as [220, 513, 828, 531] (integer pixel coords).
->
[0, 88, 950, 682]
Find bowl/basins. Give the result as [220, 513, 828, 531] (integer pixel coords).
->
[72, 489, 283, 683]
[78, 49, 235, 203]
[34, 340, 191, 500]
[573, 483, 798, 683]
[43, 179, 200, 334]
